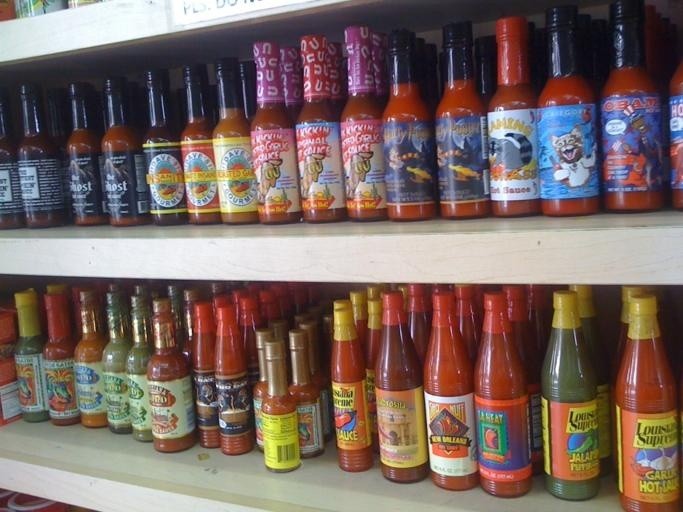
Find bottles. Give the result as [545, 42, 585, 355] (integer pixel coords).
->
[180, 63, 225, 225]
[102, 292, 135, 434]
[107, 282, 123, 293]
[71, 286, 93, 354]
[101, 74, 153, 226]
[214, 303, 255, 456]
[350, 288, 368, 368]
[141, 68, 188, 227]
[434, 20, 491, 218]
[327, 42, 347, 121]
[406, 283, 433, 362]
[247, 281, 264, 300]
[537, 5, 601, 216]
[45, 87, 71, 224]
[15, 80, 69, 229]
[166, 284, 186, 353]
[333, 298, 352, 309]
[288, 329, 326, 459]
[287, 281, 308, 315]
[124, 294, 155, 443]
[134, 284, 151, 297]
[295, 32, 347, 223]
[46, 284, 73, 300]
[374, 290, 431, 484]
[306, 282, 323, 307]
[278, 47, 304, 125]
[486, 15, 541, 216]
[0, 86, 26, 229]
[322, 313, 333, 434]
[239, 60, 257, 125]
[525, 285, 551, 358]
[191, 300, 219, 448]
[258, 288, 282, 327]
[261, 338, 301, 473]
[298, 319, 334, 443]
[427, 41, 437, 70]
[568, 284, 617, 478]
[370, 32, 392, 113]
[212, 57, 259, 224]
[268, 283, 294, 329]
[209, 281, 227, 297]
[253, 327, 276, 454]
[531, 28, 549, 96]
[14, 287, 50, 422]
[364, 298, 382, 453]
[43, 292, 81, 426]
[66, 81, 109, 226]
[613, 294, 683, 512]
[249, 40, 302, 225]
[415, 37, 438, 118]
[473, 42, 495, 105]
[268, 318, 293, 388]
[540, 290, 601, 500]
[502, 286, 544, 475]
[230, 287, 250, 328]
[229, 281, 245, 293]
[330, 308, 373, 472]
[73, 289, 109, 428]
[668, 59, 683, 210]
[211, 292, 236, 329]
[339, 24, 387, 220]
[473, 291, 534, 498]
[380, 30, 438, 220]
[598, 1, 667, 211]
[608, 284, 666, 483]
[182, 287, 204, 376]
[423, 291, 480, 490]
[366, 285, 385, 299]
[147, 312, 196, 452]
[238, 292, 264, 400]
[454, 284, 483, 363]
[438, 53, 448, 99]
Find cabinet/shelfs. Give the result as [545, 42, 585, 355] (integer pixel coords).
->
[1, 0, 682, 512]
